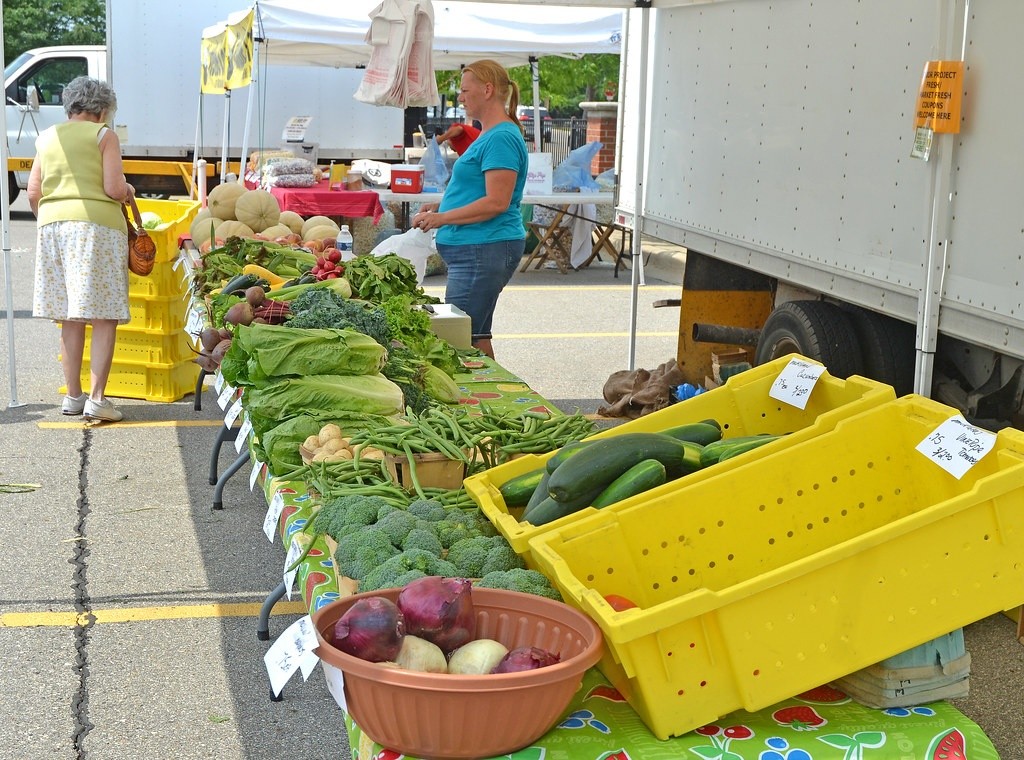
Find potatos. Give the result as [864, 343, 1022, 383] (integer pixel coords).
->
[303, 423, 384, 463]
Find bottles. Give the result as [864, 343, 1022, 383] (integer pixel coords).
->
[336, 225, 353, 262]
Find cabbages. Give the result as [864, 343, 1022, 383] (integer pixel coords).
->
[131, 212, 171, 231]
[219, 321, 405, 435]
[252, 411, 413, 477]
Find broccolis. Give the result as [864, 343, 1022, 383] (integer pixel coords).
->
[314, 495, 566, 606]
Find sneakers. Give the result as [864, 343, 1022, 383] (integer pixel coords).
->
[83, 399, 123, 421]
[62, 393, 89, 415]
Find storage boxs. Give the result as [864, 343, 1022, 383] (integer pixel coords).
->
[58, 197, 208, 402]
[390, 163, 425, 194]
[527, 393, 1023, 741]
[463, 353, 897, 591]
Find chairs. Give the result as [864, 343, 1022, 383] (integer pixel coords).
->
[519, 187, 582, 274]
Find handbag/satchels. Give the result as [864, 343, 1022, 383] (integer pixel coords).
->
[122, 184, 157, 277]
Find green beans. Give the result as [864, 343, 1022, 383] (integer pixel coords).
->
[347, 399, 612, 511]
[272, 454, 410, 573]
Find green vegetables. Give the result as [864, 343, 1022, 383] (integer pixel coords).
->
[209, 253, 440, 327]
[278, 288, 432, 415]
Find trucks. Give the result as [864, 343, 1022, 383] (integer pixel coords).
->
[606, 1, 1024, 430]
[2, 1, 439, 219]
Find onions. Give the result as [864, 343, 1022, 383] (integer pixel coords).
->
[333, 576, 563, 675]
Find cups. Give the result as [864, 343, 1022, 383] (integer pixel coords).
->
[347, 169, 363, 191]
[413, 132, 423, 149]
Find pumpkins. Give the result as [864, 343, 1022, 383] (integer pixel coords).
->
[190, 182, 340, 248]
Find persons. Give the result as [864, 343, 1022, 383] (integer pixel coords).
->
[413, 59, 529, 360]
[26, 76, 135, 421]
[436, 122, 481, 155]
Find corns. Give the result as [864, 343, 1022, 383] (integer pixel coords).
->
[193, 237, 319, 293]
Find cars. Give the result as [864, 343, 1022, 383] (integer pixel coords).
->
[447, 106, 467, 120]
[427, 107, 434, 119]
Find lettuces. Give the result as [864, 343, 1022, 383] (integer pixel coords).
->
[379, 295, 465, 403]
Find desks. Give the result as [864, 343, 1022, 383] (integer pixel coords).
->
[245, 171, 384, 225]
[177, 234, 1000, 759]
[371, 188, 613, 274]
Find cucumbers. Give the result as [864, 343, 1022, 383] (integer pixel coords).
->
[496, 419, 797, 526]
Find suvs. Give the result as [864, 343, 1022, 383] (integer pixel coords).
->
[516, 107, 553, 143]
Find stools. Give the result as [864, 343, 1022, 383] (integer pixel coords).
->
[573, 223, 626, 273]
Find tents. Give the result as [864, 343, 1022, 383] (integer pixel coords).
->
[188, 0, 646, 286]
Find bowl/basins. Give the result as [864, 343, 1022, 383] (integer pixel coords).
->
[316, 586, 606, 760]
